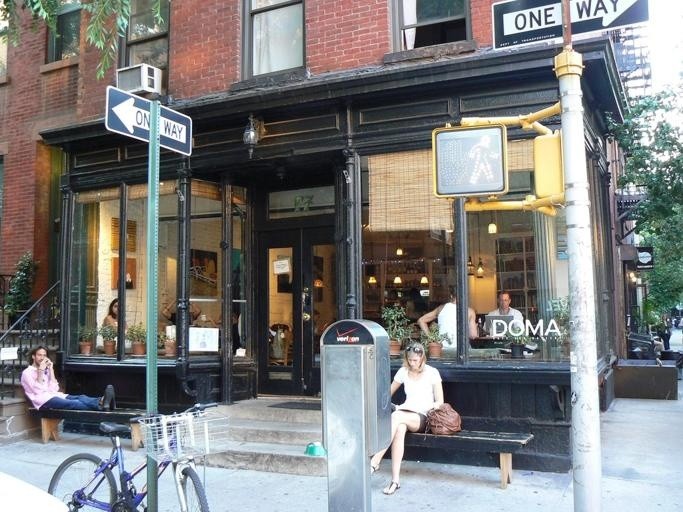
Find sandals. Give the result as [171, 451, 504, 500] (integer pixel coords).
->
[371, 465, 380, 472]
[383, 481, 401, 495]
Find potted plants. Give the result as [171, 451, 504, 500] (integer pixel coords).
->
[157, 332, 178, 357]
[554, 311, 570, 358]
[378, 303, 411, 354]
[3, 249, 41, 330]
[504, 328, 531, 359]
[420, 324, 454, 357]
[125, 321, 147, 356]
[77, 327, 99, 353]
[99, 322, 118, 355]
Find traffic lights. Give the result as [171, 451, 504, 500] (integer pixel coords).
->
[429, 122, 509, 203]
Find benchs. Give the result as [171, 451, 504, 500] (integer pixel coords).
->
[27, 406, 147, 452]
[405, 428, 535, 488]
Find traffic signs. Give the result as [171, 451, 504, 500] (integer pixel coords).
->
[491, 1, 651, 55]
[101, 84, 192, 159]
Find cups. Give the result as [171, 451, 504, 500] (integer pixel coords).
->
[200, 314, 207, 321]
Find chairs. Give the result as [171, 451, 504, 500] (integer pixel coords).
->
[268, 323, 289, 367]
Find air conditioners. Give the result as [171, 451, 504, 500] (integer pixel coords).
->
[116, 63, 161, 97]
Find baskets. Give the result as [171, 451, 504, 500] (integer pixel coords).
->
[138, 410, 231, 462]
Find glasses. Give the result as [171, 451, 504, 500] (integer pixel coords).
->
[406, 346, 423, 353]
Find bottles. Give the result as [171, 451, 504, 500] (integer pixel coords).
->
[363, 255, 445, 311]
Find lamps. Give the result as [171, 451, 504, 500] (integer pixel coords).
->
[368, 260, 377, 284]
[242, 112, 260, 161]
[396, 232, 403, 256]
[467, 211, 485, 279]
[419, 260, 429, 284]
[393, 259, 402, 284]
[488, 211, 497, 234]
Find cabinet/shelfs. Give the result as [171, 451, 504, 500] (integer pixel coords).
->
[362, 258, 433, 330]
[430, 264, 455, 319]
[487, 231, 540, 338]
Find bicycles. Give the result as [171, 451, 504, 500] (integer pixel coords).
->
[42, 397, 234, 512]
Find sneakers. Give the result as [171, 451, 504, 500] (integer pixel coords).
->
[102, 385, 116, 412]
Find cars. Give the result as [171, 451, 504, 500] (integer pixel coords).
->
[325, 409, 360, 499]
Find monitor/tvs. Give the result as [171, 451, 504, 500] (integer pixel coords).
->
[485, 315, 514, 334]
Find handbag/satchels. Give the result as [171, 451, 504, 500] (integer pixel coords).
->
[426, 403, 462, 435]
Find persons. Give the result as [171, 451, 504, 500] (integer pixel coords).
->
[482, 292, 525, 336]
[103, 298, 128, 335]
[417, 284, 478, 350]
[659, 315, 671, 350]
[390, 290, 397, 300]
[21, 345, 117, 411]
[370, 342, 444, 494]
[162, 299, 201, 325]
[406, 288, 425, 320]
